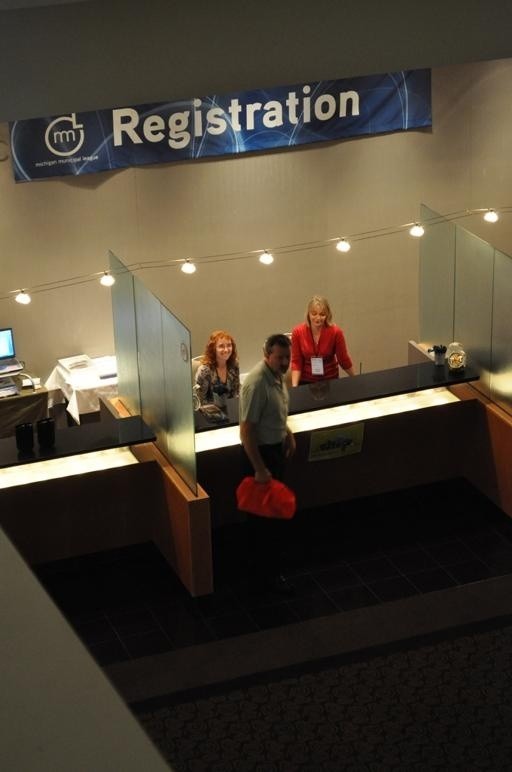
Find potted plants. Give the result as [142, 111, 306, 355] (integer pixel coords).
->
[427, 343, 449, 367]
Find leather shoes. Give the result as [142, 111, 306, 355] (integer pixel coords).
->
[266, 573, 298, 594]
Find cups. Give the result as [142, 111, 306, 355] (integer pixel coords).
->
[434, 345, 446, 366]
[37, 418, 56, 446]
[15, 423, 35, 452]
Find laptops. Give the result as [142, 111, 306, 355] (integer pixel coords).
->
[0, 327, 24, 374]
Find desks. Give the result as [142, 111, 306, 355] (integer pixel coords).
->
[44, 352, 118, 425]
[0, 372, 48, 437]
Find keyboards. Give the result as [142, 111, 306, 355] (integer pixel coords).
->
[0, 377, 19, 397]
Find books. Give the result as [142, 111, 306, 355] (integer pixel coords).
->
[57, 354, 95, 375]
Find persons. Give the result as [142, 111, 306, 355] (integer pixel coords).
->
[194, 327, 241, 405]
[232, 333, 296, 563]
[291, 294, 358, 388]
[306, 382, 332, 403]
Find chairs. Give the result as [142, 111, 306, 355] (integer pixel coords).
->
[191, 355, 205, 388]
[282, 331, 293, 390]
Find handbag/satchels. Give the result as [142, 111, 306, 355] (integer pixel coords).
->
[235, 474, 297, 520]
